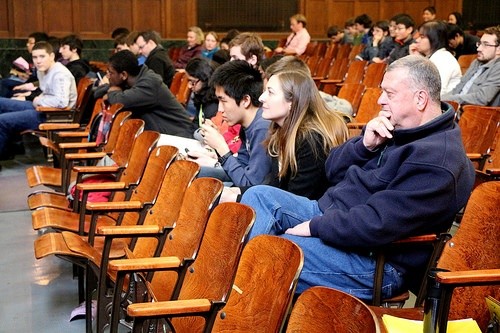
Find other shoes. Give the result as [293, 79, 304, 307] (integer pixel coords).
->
[10, 147, 25, 155]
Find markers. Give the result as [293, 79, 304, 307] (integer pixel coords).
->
[185, 148, 190, 153]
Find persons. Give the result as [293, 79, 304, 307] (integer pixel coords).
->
[253, 73, 351, 206]
[235, 52, 474, 306]
[270, 13, 310, 65]
[182, 58, 286, 197]
[90, 4, 499, 243]
[0, 42, 80, 163]
[0, 33, 87, 112]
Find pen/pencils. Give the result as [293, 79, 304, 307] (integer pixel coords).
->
[97, 72, 104, 84]
[202, 112, 205, 123]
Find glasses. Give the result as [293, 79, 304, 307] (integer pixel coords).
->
[475, 41, 498, 47]
[187, 78, 200, 86]
[27, 43, 35, 46]
[139, 43, 146, 49]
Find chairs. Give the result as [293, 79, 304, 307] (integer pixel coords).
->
[21, 36, 500, 333]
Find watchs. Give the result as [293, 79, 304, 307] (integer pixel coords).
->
[219, 151, 232, 165]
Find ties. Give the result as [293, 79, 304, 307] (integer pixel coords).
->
[286, 33, 296, 47]
